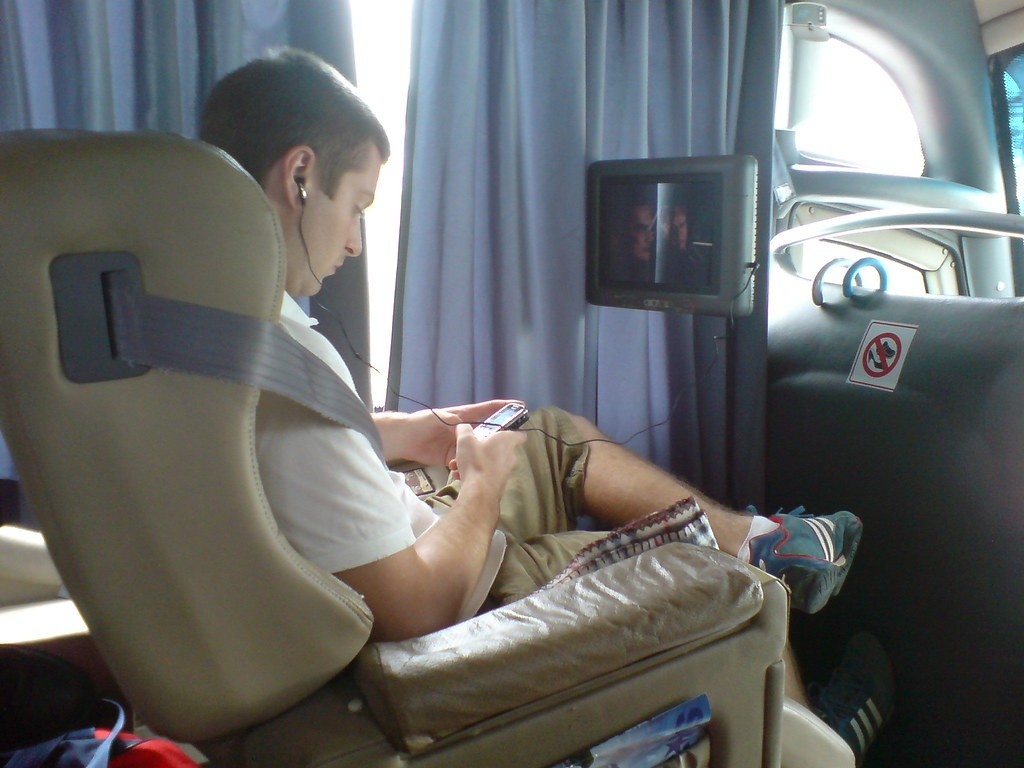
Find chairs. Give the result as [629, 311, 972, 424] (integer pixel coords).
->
[0, 126, 857, 768]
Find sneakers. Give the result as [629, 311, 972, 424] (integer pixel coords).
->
[749, 505, 863, 614]
[806, 632, 897, 768]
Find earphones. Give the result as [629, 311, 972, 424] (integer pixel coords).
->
[294, 175, 307, 204]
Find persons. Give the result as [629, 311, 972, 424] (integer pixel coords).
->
[199, 49, 897, 768]
[630, 202, 688, 263]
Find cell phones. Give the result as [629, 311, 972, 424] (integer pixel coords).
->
[473, 402, 530, 440]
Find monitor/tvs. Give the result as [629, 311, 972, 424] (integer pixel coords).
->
[585, 154, 757, 317]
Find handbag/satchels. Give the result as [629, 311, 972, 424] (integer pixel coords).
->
[0, 699, 203, 768]
